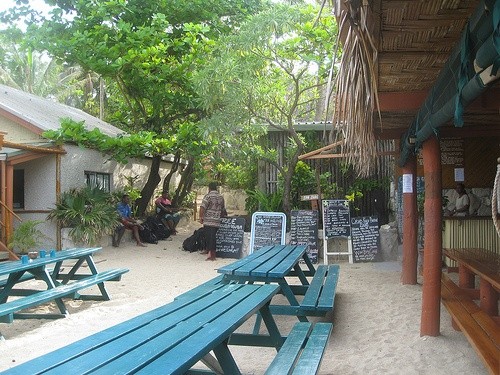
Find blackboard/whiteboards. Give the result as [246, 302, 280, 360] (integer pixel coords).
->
[397, 176, 425, 250]
[290, 210, 318, 264]
[250, 212, 286, 254]
[350, 216, 380, 262]
[215, 217, 246, 259]
[322, 200, 351, 239]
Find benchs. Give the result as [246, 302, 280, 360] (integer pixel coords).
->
[0, 268, 129, 323]
[174, 264, 340, 375]
[420, 266, 500, 375]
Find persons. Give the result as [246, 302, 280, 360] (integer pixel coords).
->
[199, 183, 225, 261]
[116, 195, 145, 246]
[450, 183, 470, 217]
[155, 191, 180, 231]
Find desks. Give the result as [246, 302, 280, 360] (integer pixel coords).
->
[0, 246, 111, 319]
[214, 244, 323, 334]
[0, 283, 294, 375]
[441, 247, 500, 317]
